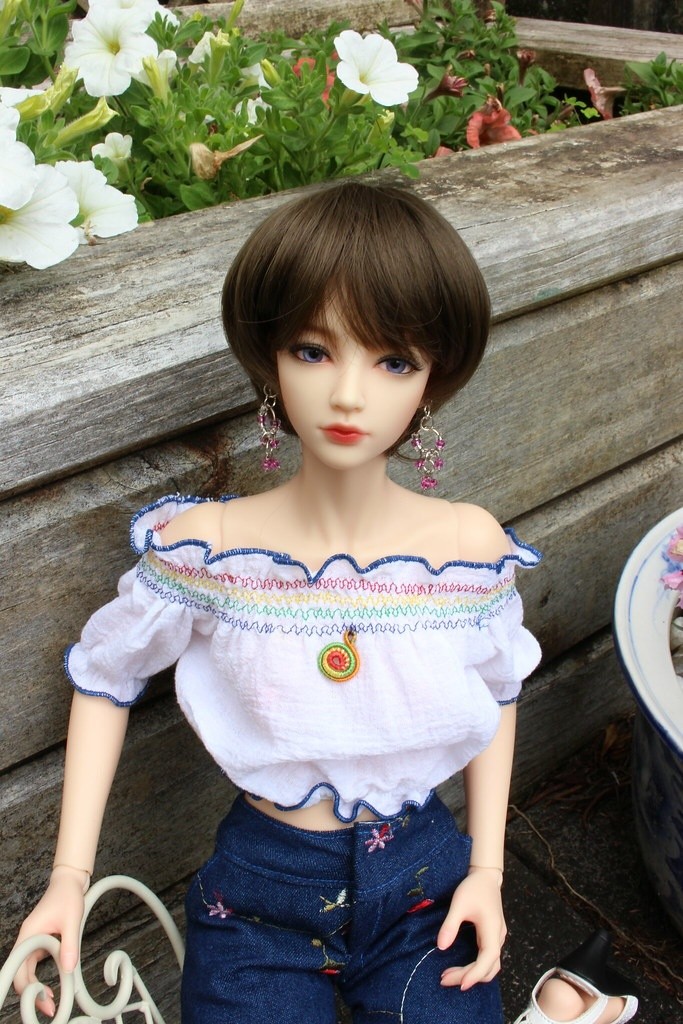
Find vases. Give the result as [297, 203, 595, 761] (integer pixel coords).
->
[614, 507, 683, 914]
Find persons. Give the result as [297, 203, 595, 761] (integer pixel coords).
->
[11, 182, 640, 1024]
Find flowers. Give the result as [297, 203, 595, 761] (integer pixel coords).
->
[658, 524, 683, 609]
